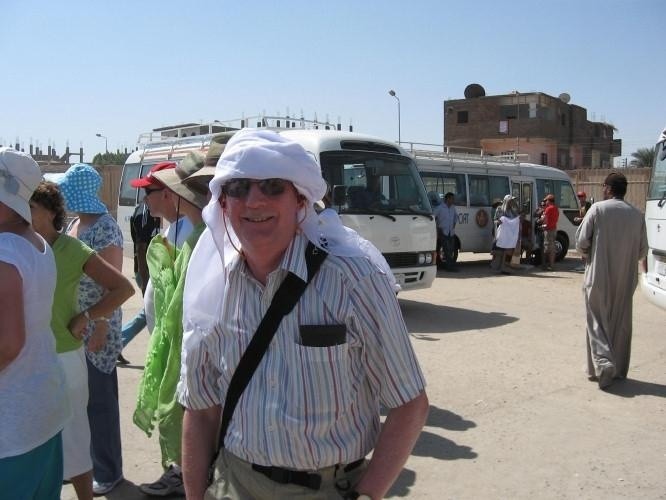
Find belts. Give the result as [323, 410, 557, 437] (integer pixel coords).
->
[250, 460, 364, 490]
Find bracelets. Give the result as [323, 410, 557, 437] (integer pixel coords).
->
[94, 315, 110, 323]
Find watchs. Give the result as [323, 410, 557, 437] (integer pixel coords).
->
[84, 310, 90, 319]
[348, 489, 372, 500]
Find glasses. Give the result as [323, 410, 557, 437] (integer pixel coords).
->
[145, 187, 164, 196]
[222, 178, 285, 198]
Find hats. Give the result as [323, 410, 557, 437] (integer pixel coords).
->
[43, 163, 108, 215]
[0, 146, 42, 227]
[491, 198, 503, 205]
[152, 150, 209, 211]
[544, 194, 554, 201]
[576, 192, 586, 197]
[180, 132, 236, 185]
[129, 161, 177, 188]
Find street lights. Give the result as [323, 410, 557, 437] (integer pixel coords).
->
[389, 90, 400, 144]
[96, 134, 107, 151]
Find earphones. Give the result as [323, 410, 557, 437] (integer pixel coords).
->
[217, 193, 308, 260]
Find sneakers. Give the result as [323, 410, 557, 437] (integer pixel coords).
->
[92, 472, 124, 494]
[139, 462, 186, 497]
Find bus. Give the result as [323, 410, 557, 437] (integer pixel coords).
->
[640, 127, 666, 307]
[117, 129, 436, 295]
[396, 141, 594, 268]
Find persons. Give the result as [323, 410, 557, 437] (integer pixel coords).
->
[133, 150, 206, 469]
[577, 192, 591, 216]
[534, 194, 559, 271]
[180, 128, 429, 500]
[0, 150, 70, 500]
[575, 172, 649, 390]
[432, 192, 462, 271]
[29, 181, 135, 500]
[533, 200, 548, 270]
[182, 134, 235, 204]
[116, 199, 160, 365]
[489, 194, 520, 274]
[130, 161, 193, 496]
[58, 162, 124, 495]
